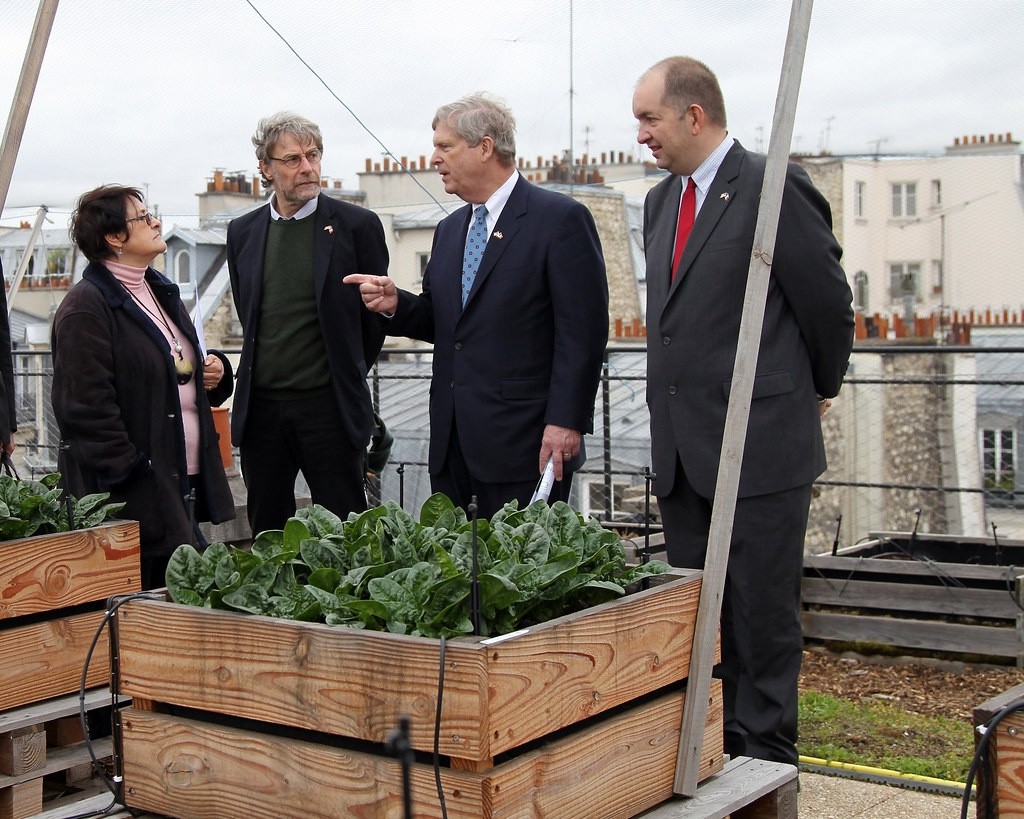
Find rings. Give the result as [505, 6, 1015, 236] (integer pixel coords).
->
[208, 385, 211, 391]
[563, 452, 571, 456]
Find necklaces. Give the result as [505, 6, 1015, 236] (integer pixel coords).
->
[118, 279, 194, 385]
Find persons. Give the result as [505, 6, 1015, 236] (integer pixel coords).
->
[0, 258, 17, 463]
[632, 56, 855, 792]
[51, 183, 236, 591]
[343, 93, 609, 525]
[224, 112, 388, 539]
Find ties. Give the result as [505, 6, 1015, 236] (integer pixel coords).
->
[670, 178, 696, 288]
[462, 205, 489, 311]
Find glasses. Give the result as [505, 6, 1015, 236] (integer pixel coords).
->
[268, 148, 324, 169]
[127, 213, 153, 227]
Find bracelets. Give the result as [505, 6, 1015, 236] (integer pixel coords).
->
[816, 397, 827, 405]
